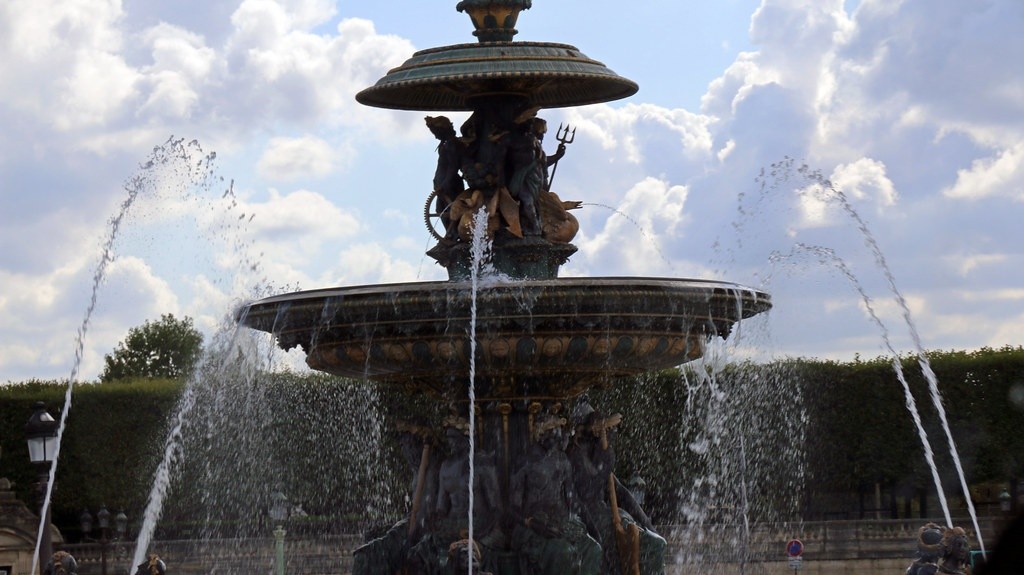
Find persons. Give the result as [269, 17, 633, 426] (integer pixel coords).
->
[905, 523, 950, 575]
[508, 116, 583, 243]
[564, 408, 666, 574]
[409, 414, 506, 574]
[425, 115, 474, 239]
[444, 100, 522, 241]
[351, 411, 445, 574]
[936, 526, 972, 575]
[508, 412, 602, 575]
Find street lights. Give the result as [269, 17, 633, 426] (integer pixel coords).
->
[80, 501, 128, 575]
[270, 484, 286, 575]
[24, 400, 61, 574]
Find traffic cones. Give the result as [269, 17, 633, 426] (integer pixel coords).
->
[998, 488, 1011, 533]
